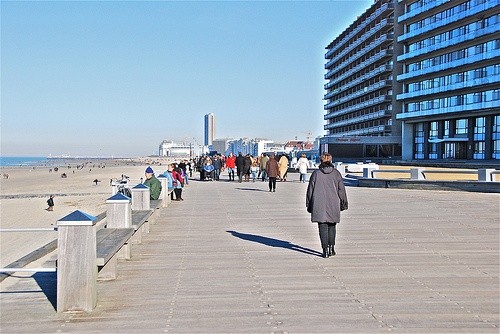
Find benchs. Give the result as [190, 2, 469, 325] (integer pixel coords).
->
[104, 210, 152, 245]
[150, 199, 163, 222]
[94, 228, 133, 282]
[168, 189, 176, 202]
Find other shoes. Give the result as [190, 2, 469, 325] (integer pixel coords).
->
[176, 197, 183, 201]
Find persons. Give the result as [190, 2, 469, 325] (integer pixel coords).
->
[265, 154, 279, 192]
[306, 153, 348, 258]
[296, 152, 309, 182]
[4, 150, 288, 201]
[46, 195, 54, 211]
[143, 166, 160, 199]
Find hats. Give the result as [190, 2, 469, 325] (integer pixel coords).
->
[146, 167, 153, 173]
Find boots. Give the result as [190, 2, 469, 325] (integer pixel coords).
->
[321, 247, 329, 258]
[329, 244, 335, 256]
